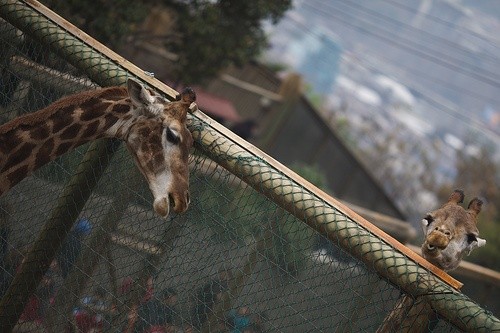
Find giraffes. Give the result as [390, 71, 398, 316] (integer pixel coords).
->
[375, 189, 487, 332]
[0, 77, 197, 219]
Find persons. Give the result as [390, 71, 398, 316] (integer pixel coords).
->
[113, 255, 160, 333]
[144, 287, 178, 333]
[184, 273, 230, 333]
[12, 260, 58, 332]
[44, 220, 93, 277]
[74, 289, 113, 333]
[224, 304, 260, 333]
